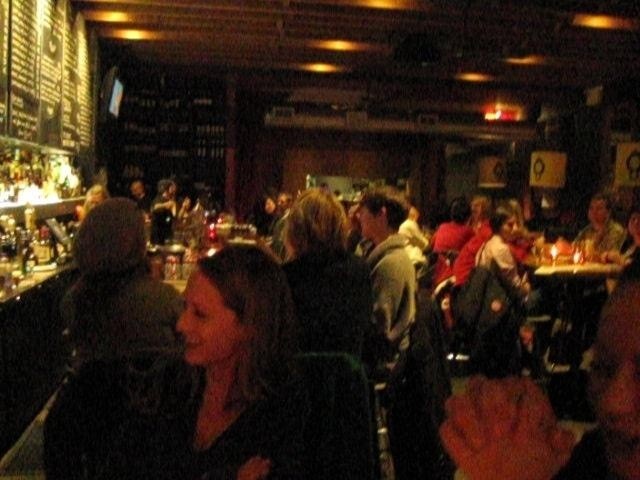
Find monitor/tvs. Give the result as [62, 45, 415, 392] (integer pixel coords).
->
[101, 65, 126, 121]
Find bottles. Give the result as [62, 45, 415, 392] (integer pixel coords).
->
[36, 226, 51, 265]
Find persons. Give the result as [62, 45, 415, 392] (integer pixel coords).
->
[51, 177, 640, 480]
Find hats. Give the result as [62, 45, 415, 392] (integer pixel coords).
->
[72, 197, 147, 277]
